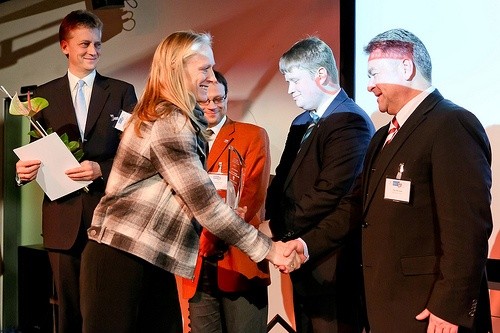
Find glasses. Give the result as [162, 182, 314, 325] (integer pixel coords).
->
[194, 93, 227, 105]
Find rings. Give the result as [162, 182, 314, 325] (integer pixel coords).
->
[290, 263, 294, 268]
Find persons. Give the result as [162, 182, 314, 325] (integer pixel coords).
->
[14, 10, 139, 333]
[79, 32, 302, 333]
[182, 69, 270, 333]
[258, 34, 376, 333]
[272, 29, 494, 333]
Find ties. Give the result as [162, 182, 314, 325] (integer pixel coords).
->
[205, 129, 214, 156]
[297, 112, 319, 154]
[382, 116, 400, 150]
[74, 79, 87, 143]
